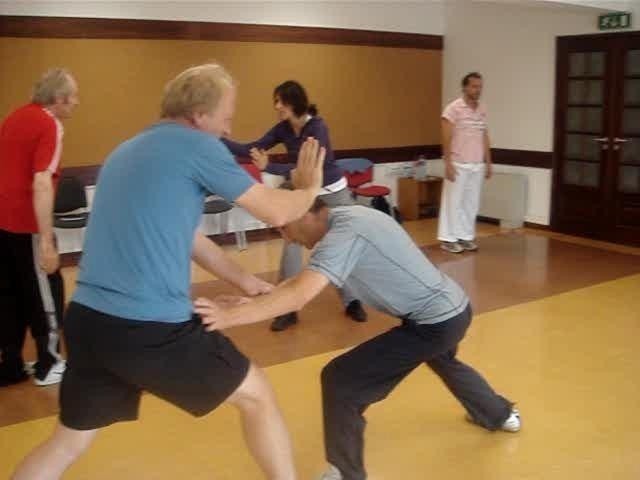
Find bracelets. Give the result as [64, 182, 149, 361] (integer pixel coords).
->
[441, 152, 450, 161]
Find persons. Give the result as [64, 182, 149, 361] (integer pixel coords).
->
[439, 73, 495, 252]
[194, 183, 522, 480]
[0, 66, 80, 388]
[222, 79, 368, 330]
[9, 65, 328, 480]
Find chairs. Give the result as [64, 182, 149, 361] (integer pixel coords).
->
[51, 176, 89, 258]
[203, 188, 232, 247]
[336, 157, 392, 211]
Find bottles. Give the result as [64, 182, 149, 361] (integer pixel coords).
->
[416, 154, 427, 178]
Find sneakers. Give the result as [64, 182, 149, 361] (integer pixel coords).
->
[465, 411, 520, 433]
[345, 300, 367, 322]
[439, 241, 479, 253]
[271, 311, 298, 330]
[24, 356, 67, 386]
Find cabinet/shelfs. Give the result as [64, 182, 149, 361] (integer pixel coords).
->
[398, 174, 444, 223]
